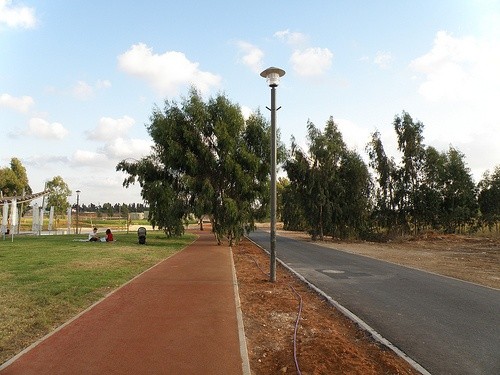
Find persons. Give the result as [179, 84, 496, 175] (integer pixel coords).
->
[105, 229, 113, 242]
[89, 228, 100, 242]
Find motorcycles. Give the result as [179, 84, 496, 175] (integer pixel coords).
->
[138, 227, 146, 244]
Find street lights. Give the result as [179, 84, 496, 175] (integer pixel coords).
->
[260, 65, 285, 280]
[75, 189, 80, 235]
